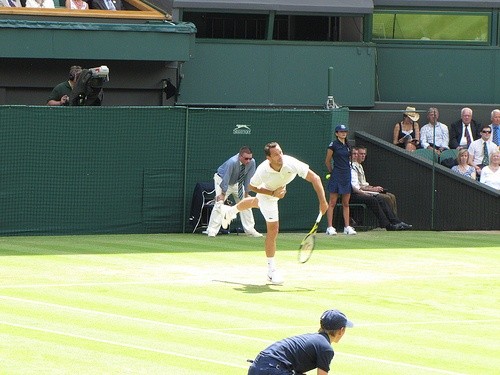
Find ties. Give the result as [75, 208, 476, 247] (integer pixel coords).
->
[464, 125, 471, 145]
[238, 164, 245, 201]
[107, 0, 114, 10]
[483, 142, 490, 166]
[8, 0, 16, 7]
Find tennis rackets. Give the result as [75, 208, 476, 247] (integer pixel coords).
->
[299, 211, 323, 264]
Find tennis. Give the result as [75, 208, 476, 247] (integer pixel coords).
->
[326, 174, 331, 180]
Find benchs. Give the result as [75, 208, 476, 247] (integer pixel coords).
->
[335, 203, 378, 232]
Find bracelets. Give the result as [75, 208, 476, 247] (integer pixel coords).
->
[272, 191, 275, 197]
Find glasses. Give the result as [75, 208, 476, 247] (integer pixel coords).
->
[353, 152, 359, 156]
[358, 153, 367, 156]
[481, 130, 491, 134]
[241, 155, 253, 160]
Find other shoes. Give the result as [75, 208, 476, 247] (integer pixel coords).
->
[386, 224, 405, 231]
[245, 228, 263, 237]
[267, 270, 284, 285]
[343, 226, 357, 235]
[326, 227, 337, 235]
[208, 232, 215, 237]
[393, 222, 413, 230]
[221, 204, 237, 229]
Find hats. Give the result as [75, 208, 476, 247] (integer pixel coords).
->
[404, 106, 419, 121]
[320, 309, 354, 330]
[334, 124, 348, 131]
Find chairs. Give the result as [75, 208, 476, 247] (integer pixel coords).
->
[440, 148, 459, 165]
[414, 149, 439, 163]
[190, 182, 239, 236]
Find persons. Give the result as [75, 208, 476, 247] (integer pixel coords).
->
[206, 146, 263, 237]
[47, 66, 102, 106]
[355, 146, 412, 230]
[220, 141, 329, 285]
[325, 125, 357, 235]
[351, 146, 404, 231]
[0, 0, 125, 10]
[393, 107, 500, 192]
[246, 308, 353, 375]
